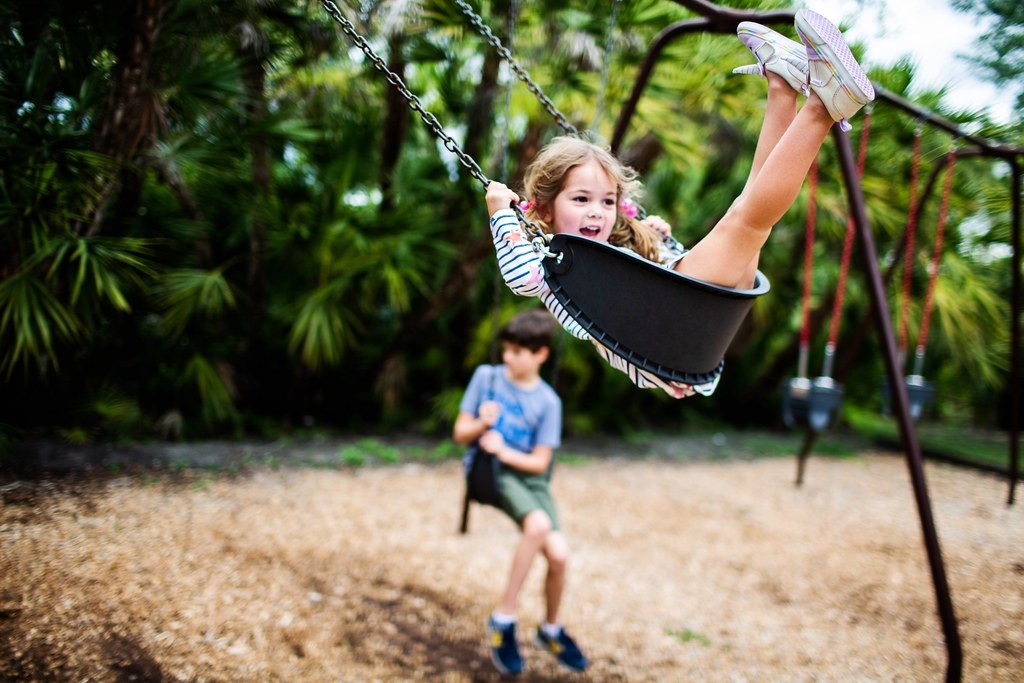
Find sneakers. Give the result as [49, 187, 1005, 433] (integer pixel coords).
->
[531, 624, 589, 673]
[730, 21, 812, 98]
[792, 7, 875, 132]
[484, 612, 524, 678]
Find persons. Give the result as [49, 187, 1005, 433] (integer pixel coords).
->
[454, 314, 587, 676]
[780, 382, 836, 487]
[487, 8, 876, 398]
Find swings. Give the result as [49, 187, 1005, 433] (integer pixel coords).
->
[319, 0, 773, 385]
[882, 121, 958, 422]
[461, 0, 623, 525]
[779, 103, 876, 434]
